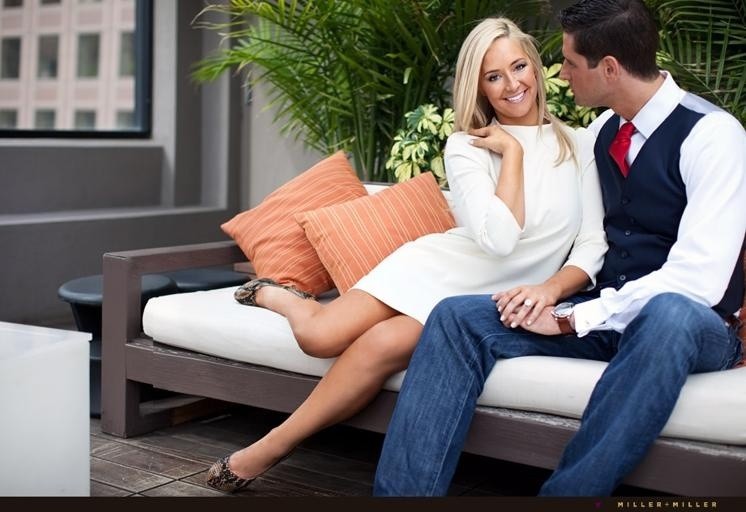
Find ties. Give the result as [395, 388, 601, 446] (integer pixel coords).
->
[606, 121, 639, 180]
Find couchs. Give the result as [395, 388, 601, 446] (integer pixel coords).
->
[97, 183, 744, 512]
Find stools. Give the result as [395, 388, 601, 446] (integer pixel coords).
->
[57, 269, 253, 418]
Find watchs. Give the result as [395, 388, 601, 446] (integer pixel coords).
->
[550, 300, 576, 335]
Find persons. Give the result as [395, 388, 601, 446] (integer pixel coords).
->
[368, 0, 746, 498]
[202, 16, 612, 496]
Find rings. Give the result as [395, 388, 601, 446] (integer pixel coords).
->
[523, 299, 531, 306]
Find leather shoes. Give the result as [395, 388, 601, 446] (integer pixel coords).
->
[206, 438, 298, 493]
[232, 276, 318, 308]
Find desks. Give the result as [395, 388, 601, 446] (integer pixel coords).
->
[0, 318, 97, 499]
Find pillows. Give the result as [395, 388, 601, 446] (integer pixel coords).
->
[220, 145, 457, 301]
